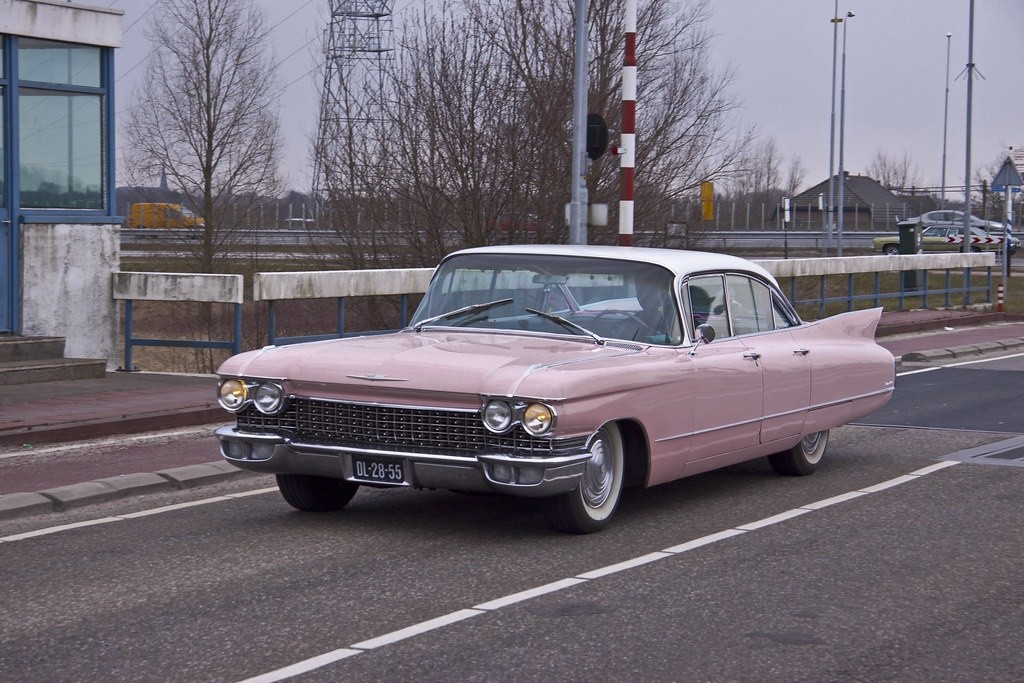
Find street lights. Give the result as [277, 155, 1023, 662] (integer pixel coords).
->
[837, 10, 856, 256]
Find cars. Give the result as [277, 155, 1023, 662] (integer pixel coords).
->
[896, 210, 1013, 238]
[214, 242, 903, 535]
[872, 225, 1022, 257]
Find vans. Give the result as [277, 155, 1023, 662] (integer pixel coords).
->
[128, 202, 205, 240]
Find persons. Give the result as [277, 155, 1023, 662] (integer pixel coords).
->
[614, 268, 702, 347]
